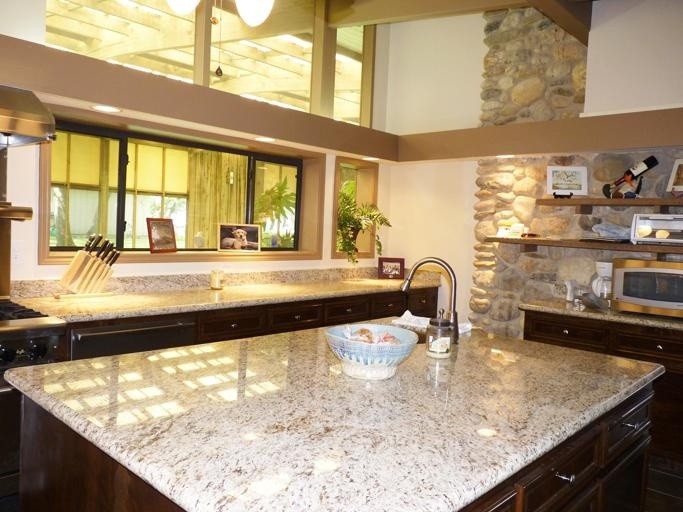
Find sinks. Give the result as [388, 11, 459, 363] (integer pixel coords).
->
[373, 322, 427, 344]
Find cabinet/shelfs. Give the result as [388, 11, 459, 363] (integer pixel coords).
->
[521, 310, 682, 463]
[196, 288, 438, 343]
[460, 381, 655, 511]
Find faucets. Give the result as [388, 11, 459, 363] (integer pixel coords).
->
[400, 257, 456, 310]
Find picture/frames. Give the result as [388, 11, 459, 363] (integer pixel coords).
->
[216, 223, 260, 252]
[146, 217, 176, 254]
[377, 257, 404, 280]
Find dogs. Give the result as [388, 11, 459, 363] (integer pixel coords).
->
[221, 229, 247, 249]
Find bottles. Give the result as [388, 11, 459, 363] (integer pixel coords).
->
[614, 155, 659, 187]
[424, 308, 454, 359]
[210, 269, 225, 290]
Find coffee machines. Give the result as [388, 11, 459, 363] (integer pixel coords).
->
[588, 261, 613, 309]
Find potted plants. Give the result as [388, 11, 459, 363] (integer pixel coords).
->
[335, 191, 392, 264]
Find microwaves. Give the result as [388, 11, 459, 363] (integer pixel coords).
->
[610, 257, 683, 318]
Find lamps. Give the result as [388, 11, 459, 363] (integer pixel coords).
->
[164, 0, 274, 78]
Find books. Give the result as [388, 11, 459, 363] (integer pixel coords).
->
[393, 309, 472, 335]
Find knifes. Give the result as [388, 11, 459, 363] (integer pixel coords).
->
[84, 233, 120, 267]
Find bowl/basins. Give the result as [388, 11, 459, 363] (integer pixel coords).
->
[325, 323, 419, 381]
